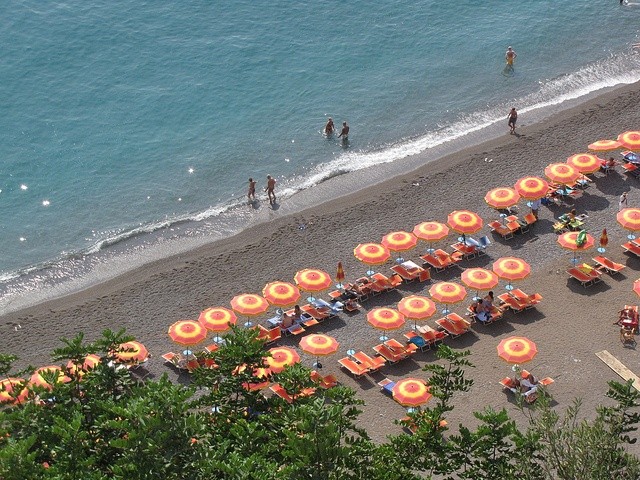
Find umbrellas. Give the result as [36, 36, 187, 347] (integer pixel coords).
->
[293, 267, 332, 303]
[617, 207, 640, 238]
[232, 361, 270, 393]
[588, 136, 622, 168]
[428, 281, 467, 314]
[366, 306, 406, 340]
[484, 186, 520, 225]
[617, 130, 640, 161]
[567, 152, 601, 176]
[398, 295, 436, 330]
[1, 340, 148, 407]
[413, 222, 449, 255]
[496, 336, 537, 372]
[261, 346, 301, 376]
[544, 162, 581, 184]
[354, 242, 389, 278]
[230, 293, 269, 327]
[198, 306, 238, 345]
[556, 230, 595, 258]
[392, 377, 432, 414]
[447, 209, 483, 243]
[262, 278, 301, 314]
[460, 267, 499, 298]
[492, 257, 531, 288]
[514, 175, 550, 201]
[299, 333, 340, 368]
[600, 227, 609, 248]
[167, 319, 207, 360]
[381, 230, 418, 265]
[335, 262, 345, 290]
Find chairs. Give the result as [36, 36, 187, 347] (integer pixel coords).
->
[507, 288, 543, 305]
[597, 157, 623, 175]
[517, 367, 555, 388]
[498, 376, 541, 405]
[457, 235, 491, 258]
[621, 304, 639, 334]
[482, 296, 512, 316]
[620, 241, 640, 258]
[566, 181, 582, 190]
[351, 351, 387, 375]
[550, 220, 571, 234]
[383, 338, 419, 360]
[327, 290, 360, 312]
[268, 382, 301, 404]
[284, 309, 320, 327]
[355, 276, 386, 297]
[377, 377, 398, 394]
[548, 182, 585, 201]
[401, 260, 431, 283]
[495, 292, 536, 316]
[402, 331, 431, 354]
[575, 171, 593, 188]
[419, 410, 448, 431]
[292, 379, 317, 398]
[576, 262, 604, 284]
[621, 161, 640, 179]
[343, 282, 372, 303]
[591, 255, 627, 276]
[417, 324, 449, 349]
[246, 324, 282, 347]
[433, 248, 463, 269]
[556, 212, 585, 231]
[371, 344, 407, 367]
[203, 343, 248, 376]
[160, 351, 201, 374]
[370, 272, 404, 293]
[486, 219, 521, 240]
[307, 370, 338, 390]
[337, 357, 370, 380]
[444, 311, 472, 336]
[182, 353, 220, 370]
[417, 253, 451, 273]
[503, 212, 537, 235]
[564, 211, 590, 222]
[434, 317, 464, 341]
[388, 265, 415, 285]
[266, 313, 306, 337]
[563, 267, 598, 289]
[449, 241, 476, 262]
[299, 304, 332, 323]
[465, 304, 500, 326]
[619, 149, 640, 165]
[631, 236, 640, 247]
[399, 415, 433, 439]
[312, 298, 344, 320]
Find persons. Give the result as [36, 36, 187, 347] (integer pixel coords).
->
[613, 306, 634, 324]
[338, 120, 350, 141]
[277, 314, 292, 329]
[505, 45, 518, 71]
[562, 209, 576, 225]
[265, 175, 277, 204]
[509, 374, 534, 392]
[290, 305, 301, 320]
[507, 107, 518, 132]
[618, 191, 628, 212]
[348, 284, 374, 295]
[469, 299, 484, 313]
[326, 117, 335, 134]
[247, 176, 256, 198]
[594, 154, 616, 167]
[530, 198, 541, 221]
[483, 291, 494, 306]
